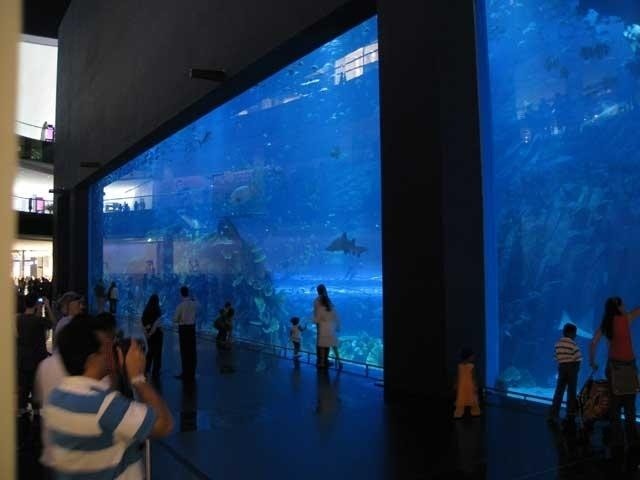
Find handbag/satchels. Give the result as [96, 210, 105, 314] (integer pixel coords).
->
[611, 365, 638, 396]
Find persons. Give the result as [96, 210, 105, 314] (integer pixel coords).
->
[213, 302, 236, 345]
[311, 284, 343, 368]
[17, 276, 175, 480]
[173, 286, 198, 380]
[289, 317, 307, 359]
[547, 323, 583, 426]
[589, 296, 640, 459]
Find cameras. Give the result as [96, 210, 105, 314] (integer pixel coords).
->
[111, 328, 146, 366]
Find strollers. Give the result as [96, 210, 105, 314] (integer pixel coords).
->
[561, 368, 611, 434]
